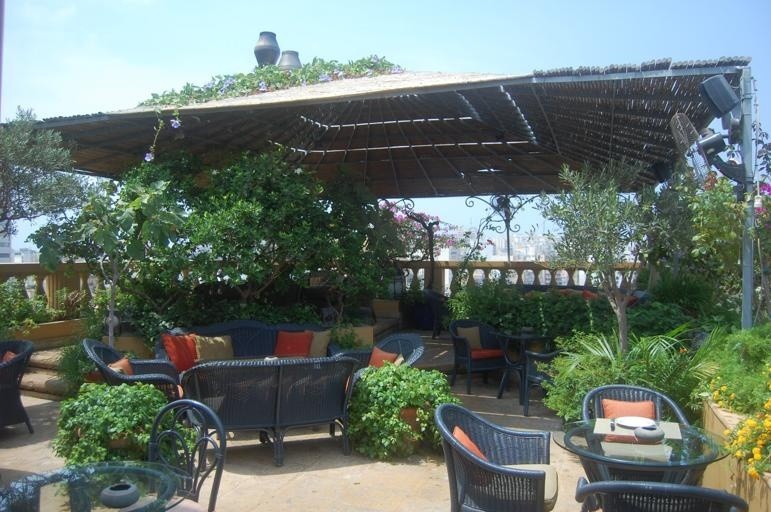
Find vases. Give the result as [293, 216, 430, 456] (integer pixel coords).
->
[276, 49, 301, 70]
[253, 30, 282, 69]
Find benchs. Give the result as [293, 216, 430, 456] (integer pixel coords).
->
[181, 354, 364, 467]
[156, 318, 340, 360]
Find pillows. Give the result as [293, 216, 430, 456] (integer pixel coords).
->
[2, 351, 17, 363]
[369, 345, 397, 372]
[193, 333, 234, 364]
[601, 398, 655, 420]
[162, 333, 197, 373]
[393, 352, 406, 368]
[452, 425, 492, 486]
[108, 352, 133, 375]
[310, 329, 333, 357]
[455, 324, 482, 348]
[274, 328, 314, 360]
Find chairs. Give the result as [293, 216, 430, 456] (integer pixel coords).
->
[523, 348, 564, 419]
[580, 382, 691, 424]
[574, 476, 750, 511]
[448, 317, 513, 395]
[148, 400, 227, 512]
[340, 331, 425, 460]
[83, 336, 181, 403]
[0, 338, 35, 443]
[433, 401, 559, 512]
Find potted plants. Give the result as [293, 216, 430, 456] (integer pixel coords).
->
[49, 381, 201, 509]
[341, 360, 463, 463]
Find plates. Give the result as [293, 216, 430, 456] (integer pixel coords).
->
[616, 413, 657, 428]
